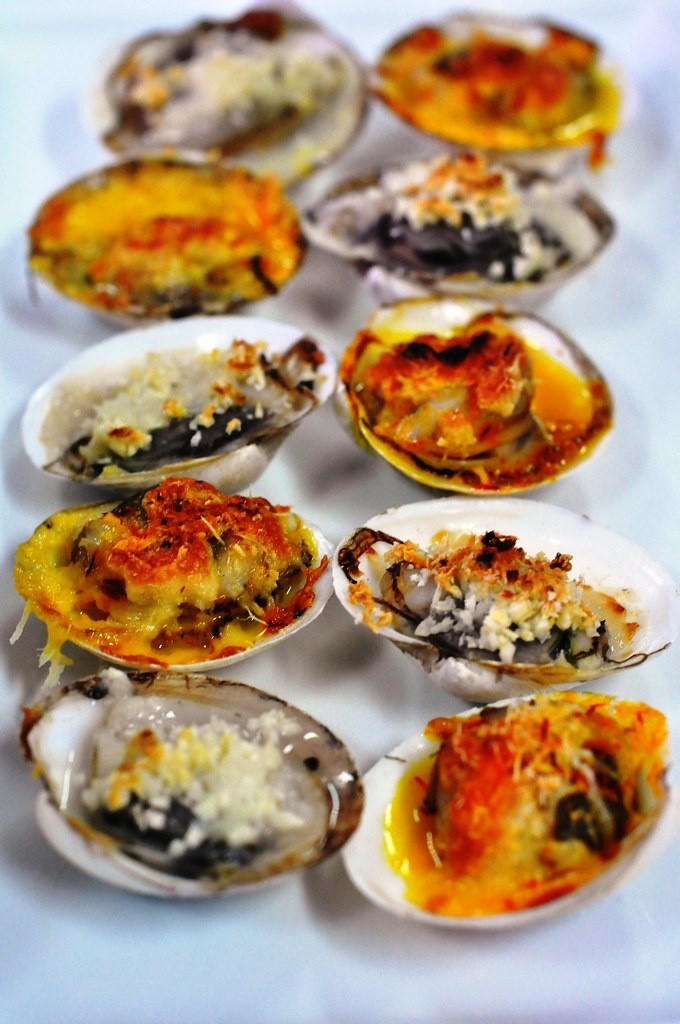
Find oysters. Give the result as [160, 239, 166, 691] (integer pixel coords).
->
[12, 0, 676, 933]
